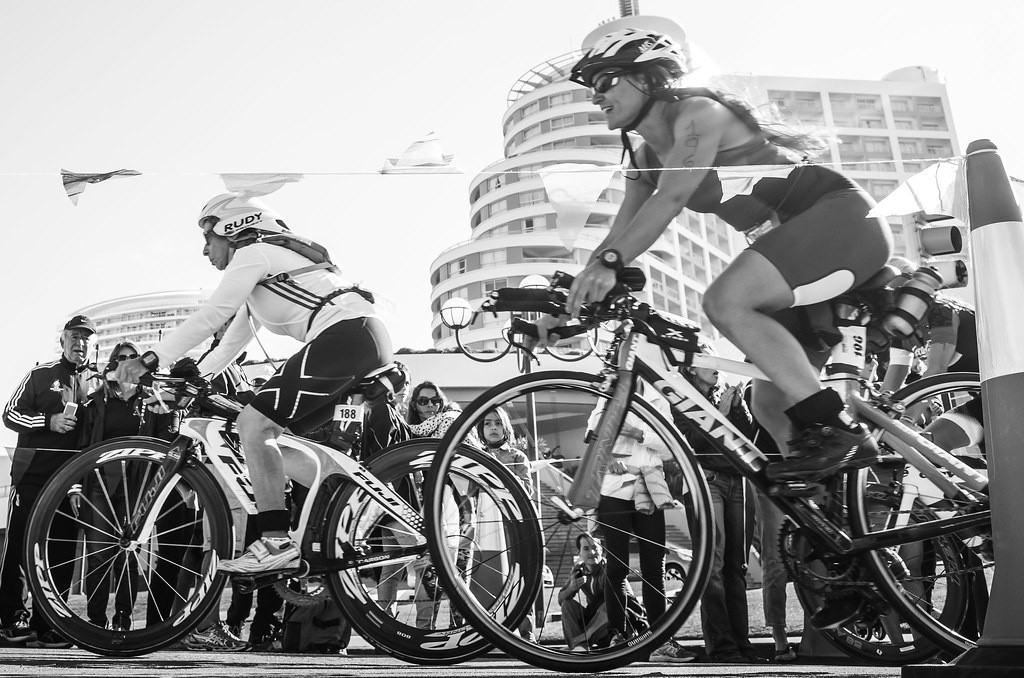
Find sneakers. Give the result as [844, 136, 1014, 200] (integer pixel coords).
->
[0, 617, 37, 643]
[764, 421, 883, 480]
[649, 638, 697, 662]
[25, 625, 74, 648]
[809, 547, 911, 632]
[216, 535, 301, 571]
[184, 618, 254, 652]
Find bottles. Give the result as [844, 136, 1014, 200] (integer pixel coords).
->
[574, 564, 584, 579]
[883, 266, 942, 338]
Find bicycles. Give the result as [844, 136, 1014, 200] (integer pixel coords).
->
[22, 267, 998, 674]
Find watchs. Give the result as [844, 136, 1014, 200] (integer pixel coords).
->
[140, 350, 159, 371]
[595, 248, 625, 275]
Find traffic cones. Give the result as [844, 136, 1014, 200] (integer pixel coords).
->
[894, 135, 1024, 678]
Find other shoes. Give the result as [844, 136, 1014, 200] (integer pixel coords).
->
[524, 630, 537, 645]
[724, 654, 769, 663]
[91, 614, 132, 632]
[228, 619, 247, 639]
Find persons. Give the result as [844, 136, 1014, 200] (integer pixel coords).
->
[522, 25, 897, 633]
[361, 254, 987, 664]
[0, 314, 292, 652]
[111, 192, 394, 574]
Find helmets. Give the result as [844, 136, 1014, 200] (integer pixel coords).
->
[568, 28, 689, 88]
[198, 193, 293, 237]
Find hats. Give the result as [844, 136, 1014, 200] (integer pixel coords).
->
[64, 315, 96, 334]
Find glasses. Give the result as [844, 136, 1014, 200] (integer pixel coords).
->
[111, 353, 141, 361]
[589, 67, 639, 96]
[415, 395, 441, 406]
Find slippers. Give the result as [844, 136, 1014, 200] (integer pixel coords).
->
[774, 644, 798, 664]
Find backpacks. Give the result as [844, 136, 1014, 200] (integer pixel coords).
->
[280, 578, 353, 656]
[255, 229, 342, 287]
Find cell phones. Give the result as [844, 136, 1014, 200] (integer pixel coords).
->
[573, 567, 583, 578]
[63, 401, 78, 415]
